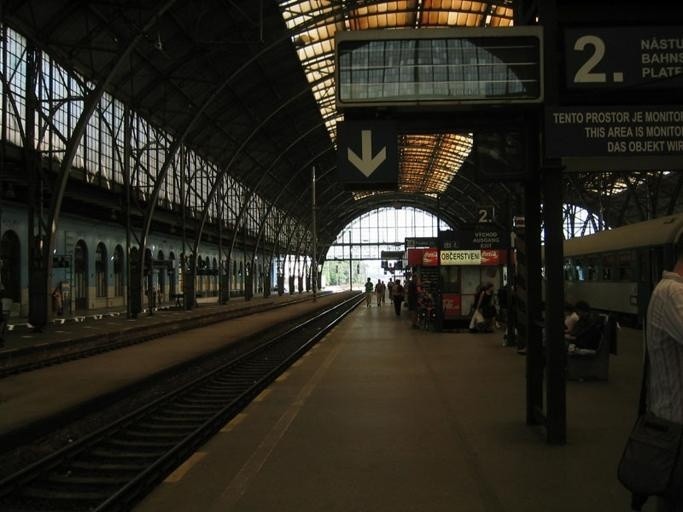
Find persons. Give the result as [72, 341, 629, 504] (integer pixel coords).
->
[640, 232, 683, 512]
[365, 275, 420, 329]
[52, 288, 61, 318]
[473, 283, 599, 368]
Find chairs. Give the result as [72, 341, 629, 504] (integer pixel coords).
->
[565, 313, 608, 382]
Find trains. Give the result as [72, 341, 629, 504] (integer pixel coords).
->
[541, 213, 683, 328]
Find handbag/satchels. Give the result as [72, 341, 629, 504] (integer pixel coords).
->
[617, 409, 683, 499]
